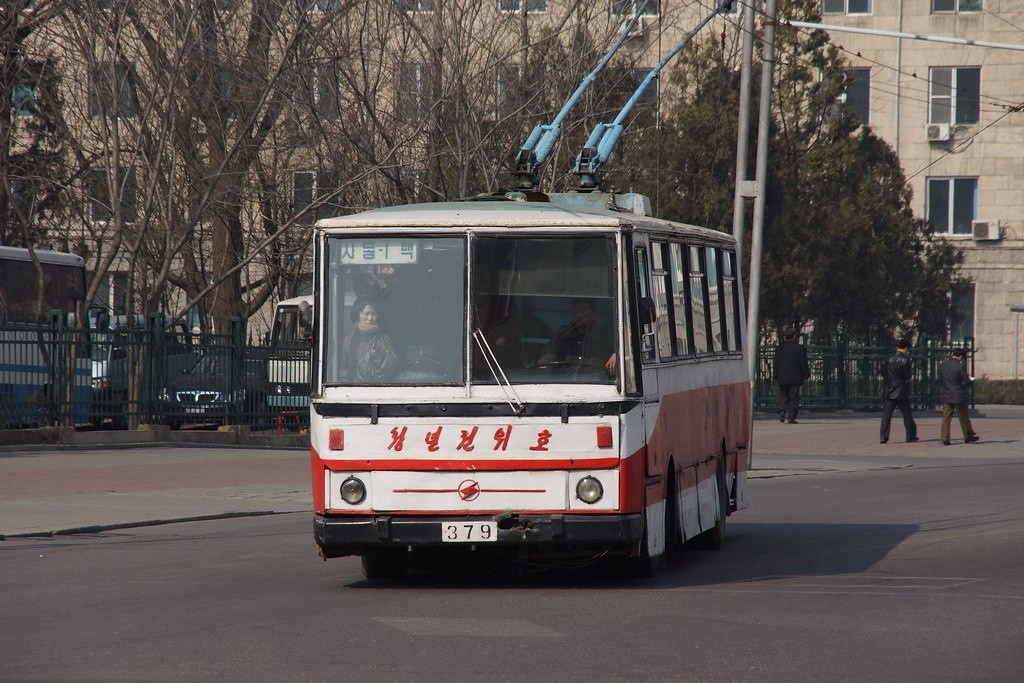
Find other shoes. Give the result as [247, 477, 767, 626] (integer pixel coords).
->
[906, 437, 918, 442]
[943, 440, 951, 445]
[881, 438, 886, 443]
[779, 412, 785, 422]
[966, 435, 979, 443]
[789, 419, 797, 424]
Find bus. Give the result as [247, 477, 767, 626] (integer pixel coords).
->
[265, 293, 313, 432]
[305, 0, 753, 587]
[0, 244, 111, 429]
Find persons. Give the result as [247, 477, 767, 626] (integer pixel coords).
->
[773, 328, 810, 424]
[384, 266, 461, 365]
[474, 271, 519, 382]
[940, 348, 979, 445]
[880, 339, 919, 444]
[537, 294, 618, 376]
[336, 295, 399, 384]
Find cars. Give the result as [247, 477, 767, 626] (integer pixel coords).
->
[154, 345, 269, 431]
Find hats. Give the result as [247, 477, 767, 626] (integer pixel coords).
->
[782, 327, 795, 338]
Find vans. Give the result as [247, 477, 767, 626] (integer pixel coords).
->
[91, 313, 203, 427]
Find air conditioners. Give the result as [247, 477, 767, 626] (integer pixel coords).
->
[926, 123, 949, 142]
[614, 15, 642, 37]
[972, 221, 1003, 241]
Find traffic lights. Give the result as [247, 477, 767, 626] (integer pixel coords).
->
[290, 171, 318, 227]
[316, 168, 340, 222]
